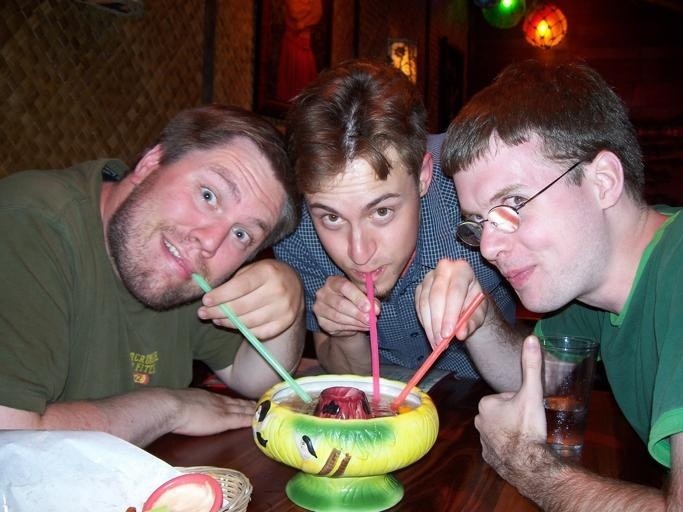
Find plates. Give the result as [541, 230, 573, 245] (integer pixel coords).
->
[142, 473, 222, 512]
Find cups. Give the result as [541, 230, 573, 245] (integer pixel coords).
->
[537, 335, 600, 457]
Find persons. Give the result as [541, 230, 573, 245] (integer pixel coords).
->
[414, 59, 683, 512]
[1, 105, 306, 448]
[274, 56, 514, 378]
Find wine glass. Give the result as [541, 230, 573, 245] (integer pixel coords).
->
[251, 374, 440, 512]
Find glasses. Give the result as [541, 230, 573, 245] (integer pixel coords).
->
[455, 159, 593, 247]
